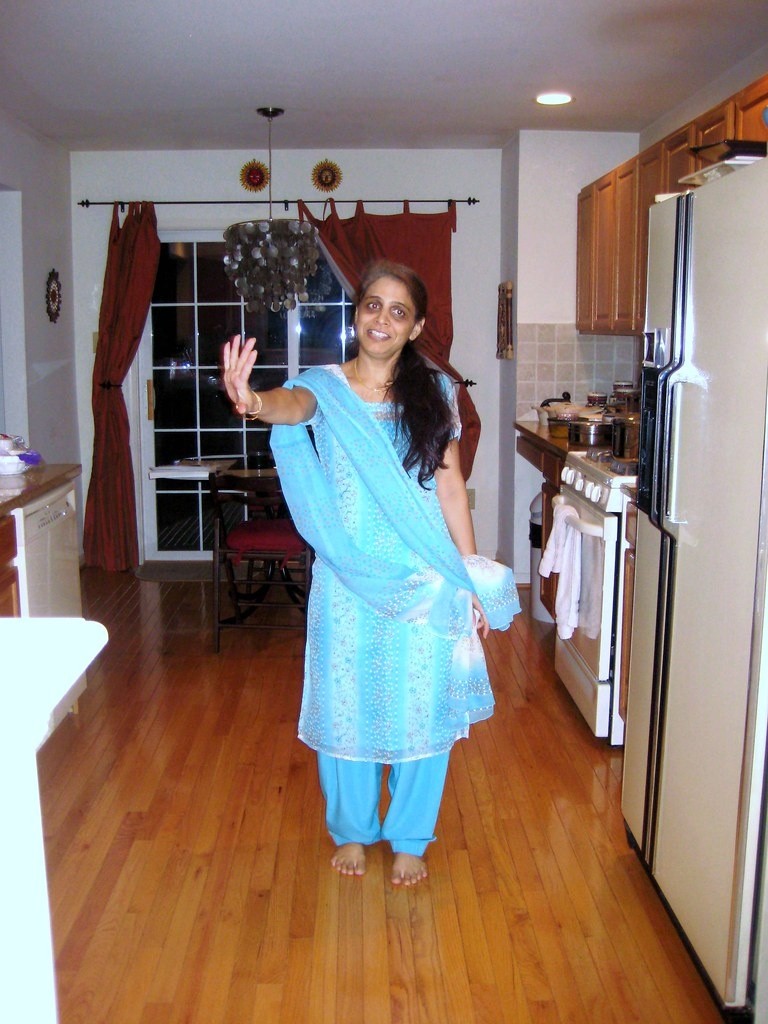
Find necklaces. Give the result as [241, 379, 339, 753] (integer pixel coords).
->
[354, 359, 395, 392]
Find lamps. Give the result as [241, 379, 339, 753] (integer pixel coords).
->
[223, 107, 320, 313]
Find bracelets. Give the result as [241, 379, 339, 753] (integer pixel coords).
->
[240, 391, 262, 421]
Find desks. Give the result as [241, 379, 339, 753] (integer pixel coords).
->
[0, 617, 109, 1024]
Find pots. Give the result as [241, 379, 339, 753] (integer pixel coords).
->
[612, 417, 640, 462]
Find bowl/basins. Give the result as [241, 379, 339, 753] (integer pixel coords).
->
[568, 421, 613, 446]
[547, 416, 589, 438]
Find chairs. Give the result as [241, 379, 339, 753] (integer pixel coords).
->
[208, 472, 314, 650]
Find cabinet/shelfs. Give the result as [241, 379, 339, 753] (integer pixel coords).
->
[0, 464, 88, 715]
[512, 421, 612, 620]
[618, 482, 636, 748]
[576, 73, 768, 335]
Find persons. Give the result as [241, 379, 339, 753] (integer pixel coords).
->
[221, 262, 490, 888]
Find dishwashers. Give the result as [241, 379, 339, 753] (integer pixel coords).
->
[6, 482, 82, 619]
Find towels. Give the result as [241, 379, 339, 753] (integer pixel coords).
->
[537, 502, 580, 641]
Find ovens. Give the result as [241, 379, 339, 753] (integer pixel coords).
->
[552, 485, 625, 745]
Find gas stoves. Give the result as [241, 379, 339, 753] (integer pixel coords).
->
[561, 450, 638, 513]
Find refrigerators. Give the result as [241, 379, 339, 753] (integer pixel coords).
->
[622, 158, 768, 1024]
